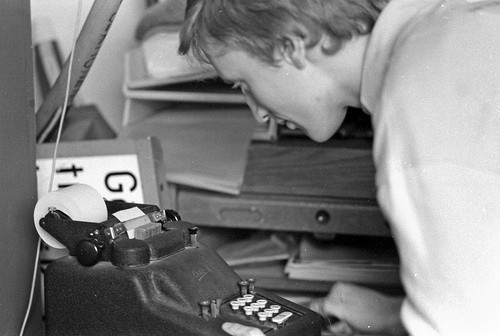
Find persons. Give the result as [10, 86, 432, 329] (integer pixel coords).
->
[177, 0, 500, 336]
